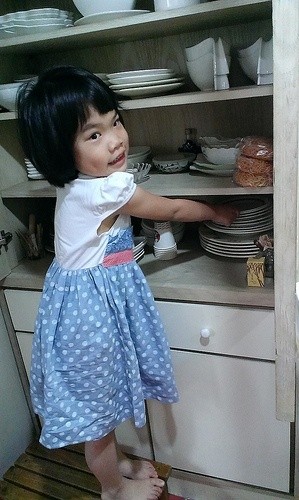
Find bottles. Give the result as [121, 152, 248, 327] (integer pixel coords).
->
[182, 128, 198, 156]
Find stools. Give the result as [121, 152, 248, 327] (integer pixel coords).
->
[0, 440, 170, 500]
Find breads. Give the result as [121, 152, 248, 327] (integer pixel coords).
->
[233, 136, 273, 187]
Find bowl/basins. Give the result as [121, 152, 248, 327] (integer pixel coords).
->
[200, 147, 240, 165]
[185, 37, 230, 92]
[127, 163, 151, 183]
[73, 0, 134, 17]
[0, 82, 24, 112]
[128, 145, 151, 167]
[140, 219, 184, 247]
[152, 153, 195, 173]
[238, 36, 273, 85]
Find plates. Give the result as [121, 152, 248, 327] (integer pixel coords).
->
[190, 164, 235, 177]
[0, 8, 73, 38]
[198, 136, 242, 149]
[193, 160, 241, 169]
[92, 68, 185, 100]
[74, 9, 150, 26]
[199, 195, 274, 258]
[24, 158, 45, 180]
[133, 236, 148, 262]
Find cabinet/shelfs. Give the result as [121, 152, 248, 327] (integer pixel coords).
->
[0, 0, 297, 495]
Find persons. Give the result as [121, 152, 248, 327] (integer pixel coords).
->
[15, 66, 240, 500]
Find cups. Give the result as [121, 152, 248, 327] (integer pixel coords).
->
[154, 221, 178, 260]
[153, 0, 200, 12]
[20, 235, 44, 261]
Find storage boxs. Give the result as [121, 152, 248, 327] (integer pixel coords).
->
[247, 256, 265, 287]
